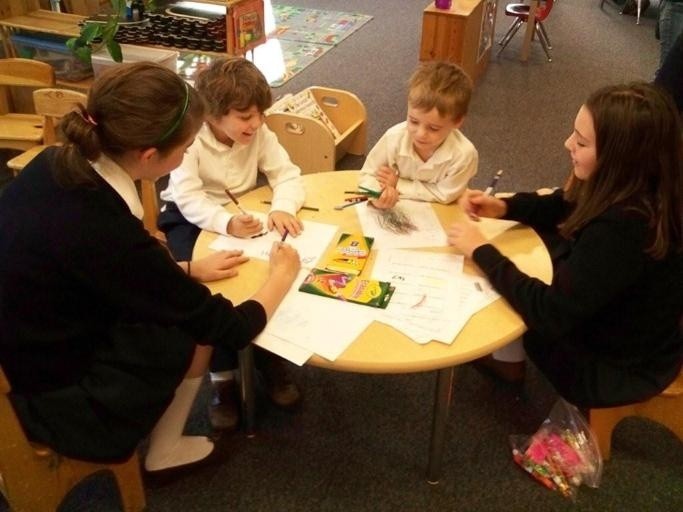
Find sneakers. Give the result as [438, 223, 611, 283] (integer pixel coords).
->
[250, 355, 302, 410]
[207, 376, 244, 431]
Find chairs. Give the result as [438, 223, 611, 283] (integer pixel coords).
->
[0, 55, 93, 180]
[0, 372, 150, 512]
[491, 1, 558, 65]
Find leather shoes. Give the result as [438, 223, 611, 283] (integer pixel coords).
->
[471, 353, 528, 385]
[140, 436, 233, 488]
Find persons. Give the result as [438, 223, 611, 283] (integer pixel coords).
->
[2, 60, 300, 483]
[449, 82, 682, 408]
[359, 63, 479, 212]
[156, 55, 306, 431]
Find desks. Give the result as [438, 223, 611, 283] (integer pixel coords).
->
[186, 170, 556, 486]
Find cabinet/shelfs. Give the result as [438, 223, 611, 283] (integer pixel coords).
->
[422, 0, 499, 85]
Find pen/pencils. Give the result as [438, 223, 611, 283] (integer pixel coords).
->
[280, 230, 288, 248]
[473, 170, 504, 214]
[344, 186, 403, 202]
[225, 190, 246, 214]
[260, 201, 318, 211]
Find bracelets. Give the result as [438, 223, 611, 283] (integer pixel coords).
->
[186, 261, 190, 275]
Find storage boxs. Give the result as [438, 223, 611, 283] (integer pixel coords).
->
[13, 34, 182, 94]
